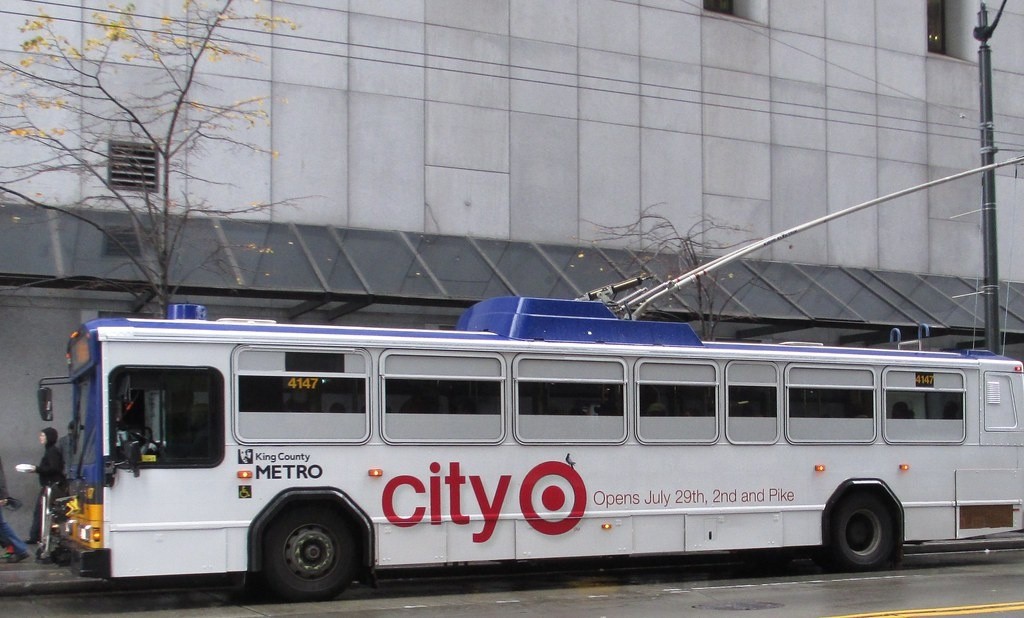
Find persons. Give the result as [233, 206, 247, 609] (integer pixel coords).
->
[25, 427, 70, 544]
[184, 403, 212, 462]
[58, 420, 85, 480]
[0, 458, 29, 563]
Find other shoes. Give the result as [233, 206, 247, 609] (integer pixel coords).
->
[2, 552, 13, 558]
[24, 538, 40, 544]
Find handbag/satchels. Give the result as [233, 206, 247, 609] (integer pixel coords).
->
[7, 552, 31, 563]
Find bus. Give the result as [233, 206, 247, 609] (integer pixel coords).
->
[33, 140, 1024, 606]
